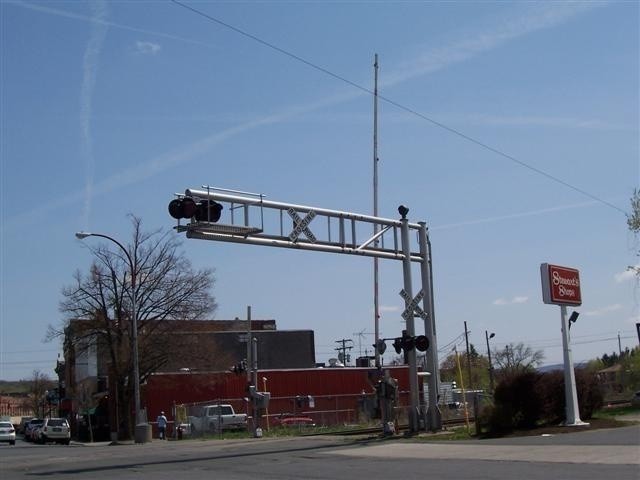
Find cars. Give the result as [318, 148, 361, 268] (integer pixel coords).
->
[0, 423, 16, 444]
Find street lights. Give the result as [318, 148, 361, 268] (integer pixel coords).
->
[76, 231, 141, 423]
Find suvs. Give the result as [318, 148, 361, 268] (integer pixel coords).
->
[25, 417, 72, 445]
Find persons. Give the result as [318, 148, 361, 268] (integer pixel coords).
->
[156, 411, 168, 440]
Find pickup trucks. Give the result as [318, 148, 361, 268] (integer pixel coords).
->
[188, 403, 247, 437]
